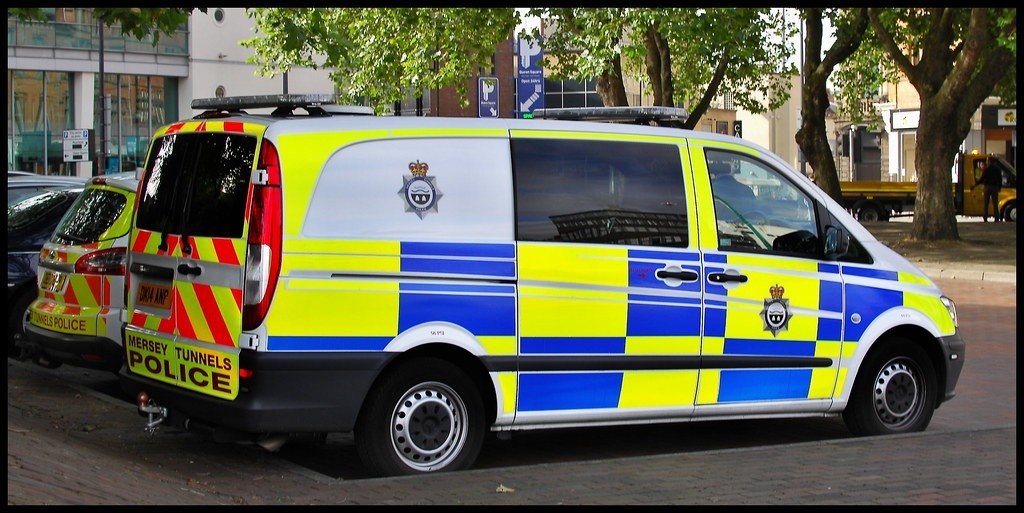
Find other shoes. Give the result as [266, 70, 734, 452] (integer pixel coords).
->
[995, 217, 999, 222]
[983, 217, 986, 222]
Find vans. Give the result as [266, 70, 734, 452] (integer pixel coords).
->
[14, 167, 142, 373]
[736, 179, 810, 221]
[118, 92, 964, 476]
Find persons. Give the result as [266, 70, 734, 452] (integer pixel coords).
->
[805, 174, 816, 234]
[712, 160, 759, 224]
[970, 157, 1002, 222]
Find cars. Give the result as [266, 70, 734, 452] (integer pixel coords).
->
[9, 184, 84, 344]
[127, 137, 151, 160]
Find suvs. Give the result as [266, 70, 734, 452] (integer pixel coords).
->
[8, 170, 88, 207]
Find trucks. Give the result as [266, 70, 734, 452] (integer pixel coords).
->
[839, 149, 1016, 223]
